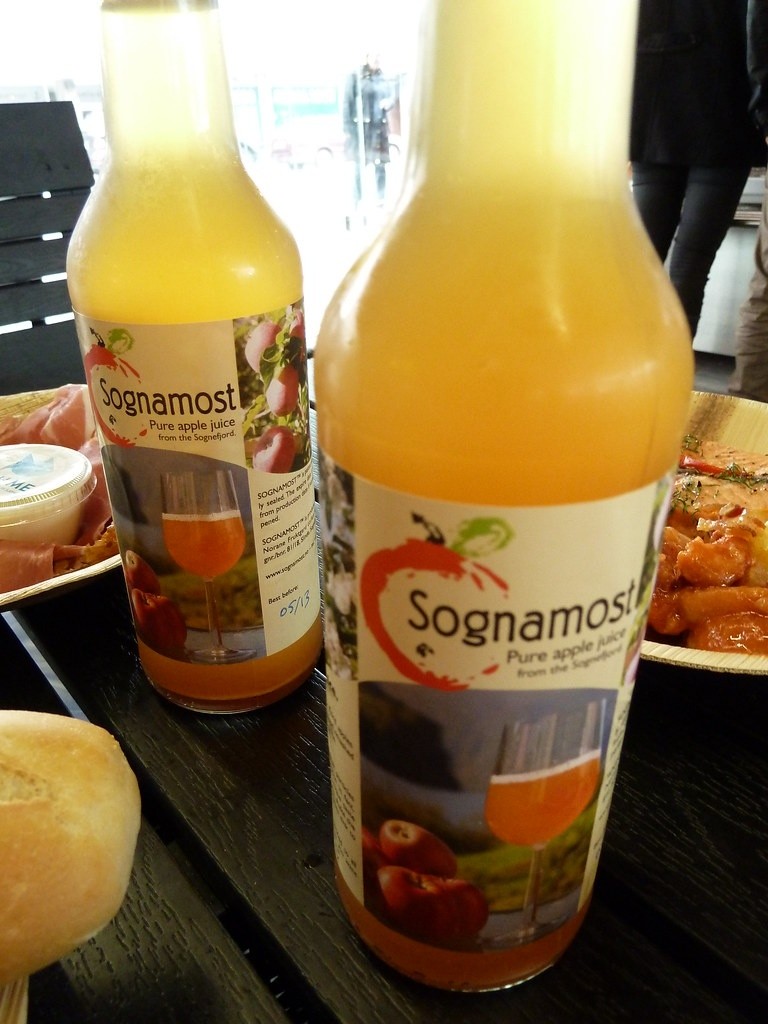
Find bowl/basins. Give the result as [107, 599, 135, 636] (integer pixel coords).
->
[0, 444, 98, 545]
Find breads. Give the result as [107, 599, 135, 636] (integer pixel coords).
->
[0, 708, 141, 987]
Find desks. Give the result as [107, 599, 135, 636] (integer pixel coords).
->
[0, 567, 768, 1024]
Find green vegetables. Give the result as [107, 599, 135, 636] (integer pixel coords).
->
[669, 433, 768, 531]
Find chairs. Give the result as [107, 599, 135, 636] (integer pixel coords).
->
[0, 99, 96, 396]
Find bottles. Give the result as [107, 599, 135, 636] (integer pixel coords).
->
[314, 0, 694, 993]
[66, 0, 321, 714]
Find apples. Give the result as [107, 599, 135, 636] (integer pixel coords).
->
[123, 549, 187, 658]
[246, 310, 308, 475]
[359, 819, 488, 951]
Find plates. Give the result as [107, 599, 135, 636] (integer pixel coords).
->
[639, 390, 768, 673]
[0, 387, 123, 607]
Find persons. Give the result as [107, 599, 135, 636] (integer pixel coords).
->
[343, 50, 394, 199]
[629, 0, 768, 402]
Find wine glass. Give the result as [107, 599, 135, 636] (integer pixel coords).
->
[161, 470, 258, 663]
[484, 697, 606, 945]
